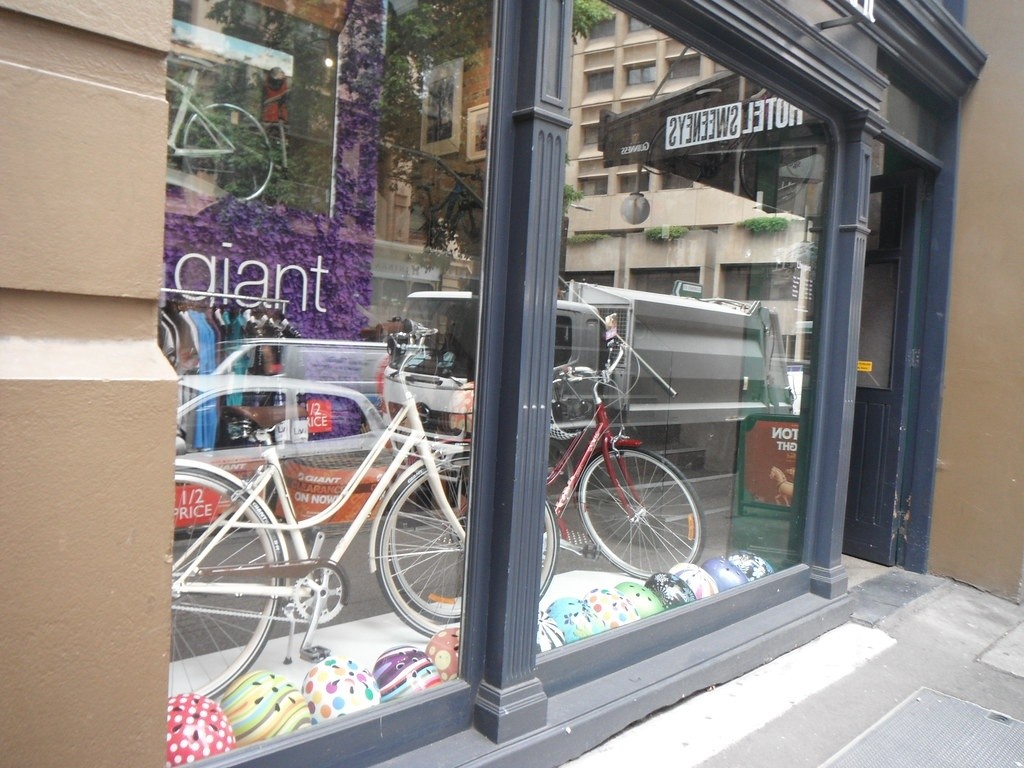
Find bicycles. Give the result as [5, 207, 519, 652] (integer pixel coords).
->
[166, 53, 276, 205]
[170, 321, 709, 704]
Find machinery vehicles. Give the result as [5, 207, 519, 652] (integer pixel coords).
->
[380, 280, 792, 505]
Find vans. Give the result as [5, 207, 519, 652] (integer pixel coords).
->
[172, 337, 397, 491]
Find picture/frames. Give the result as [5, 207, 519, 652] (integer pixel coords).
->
[418, 55, 465, 161]
[465, 100, 494, 164]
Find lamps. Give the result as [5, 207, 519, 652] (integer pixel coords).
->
[620, 164, 652, 225]
